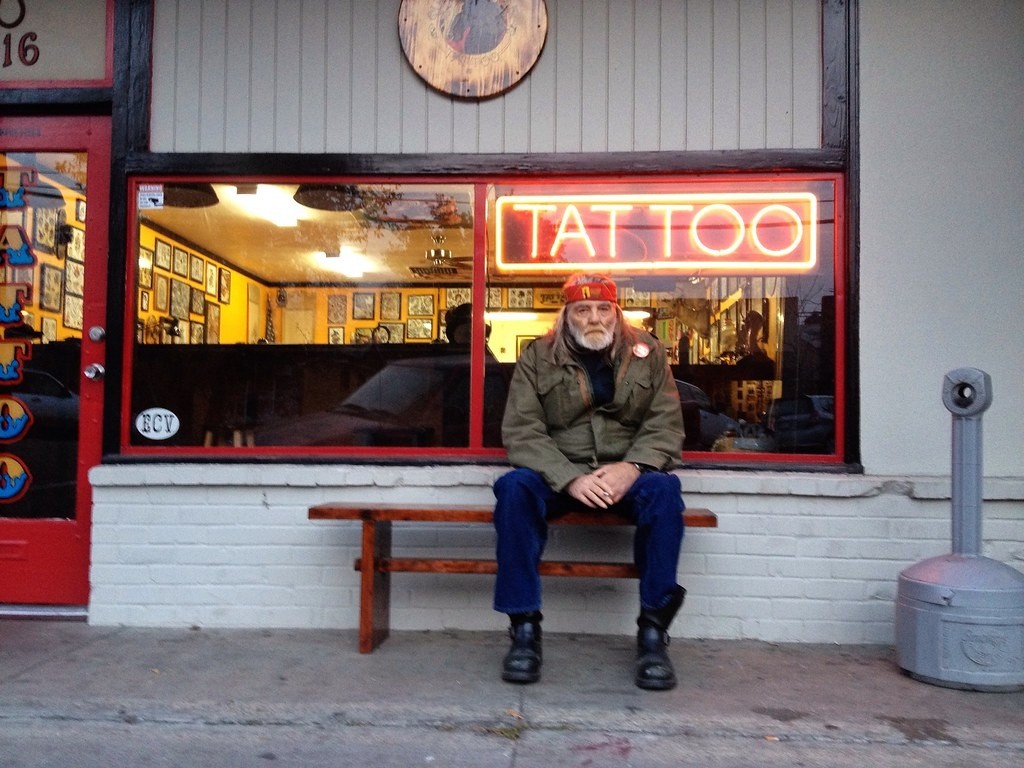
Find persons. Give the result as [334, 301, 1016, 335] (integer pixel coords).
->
[436, 303, 509, 447]
[492, 272, 687, 691]
[671, 327, 695, 365]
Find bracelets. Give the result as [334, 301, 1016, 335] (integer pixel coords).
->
[633, 462, 642, 472]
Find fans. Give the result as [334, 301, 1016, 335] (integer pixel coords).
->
[412, 238, 473, 272]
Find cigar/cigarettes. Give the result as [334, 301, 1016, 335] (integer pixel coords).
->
[764, 736, 779, 741]
[834, 641, 839, 645]
[603, 492, 609, 496]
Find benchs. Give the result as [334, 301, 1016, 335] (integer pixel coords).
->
[308, 501, 718, 655]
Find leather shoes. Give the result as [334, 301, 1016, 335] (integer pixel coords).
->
[633, 627, 676, 689]
[501, 622, 542, 682]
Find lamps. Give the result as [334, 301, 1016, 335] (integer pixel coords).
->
[164, 181, 219, 208]
[293, 184, 364, 211]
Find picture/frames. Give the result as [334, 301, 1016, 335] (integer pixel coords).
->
[352, 292, 375, 320]
[355, 328, 375, 343]
[408, 295, 434, 316]
[379, 322, 405, 344]
[515, 334, 540, 361]
[328, 326, 345, 344]
[439, 287, 502, 342]
[380, 292, 401, 320]
[33, 198, 233, 345]
[406, 318, 432, 339]
[507, 287, 534, 308]
